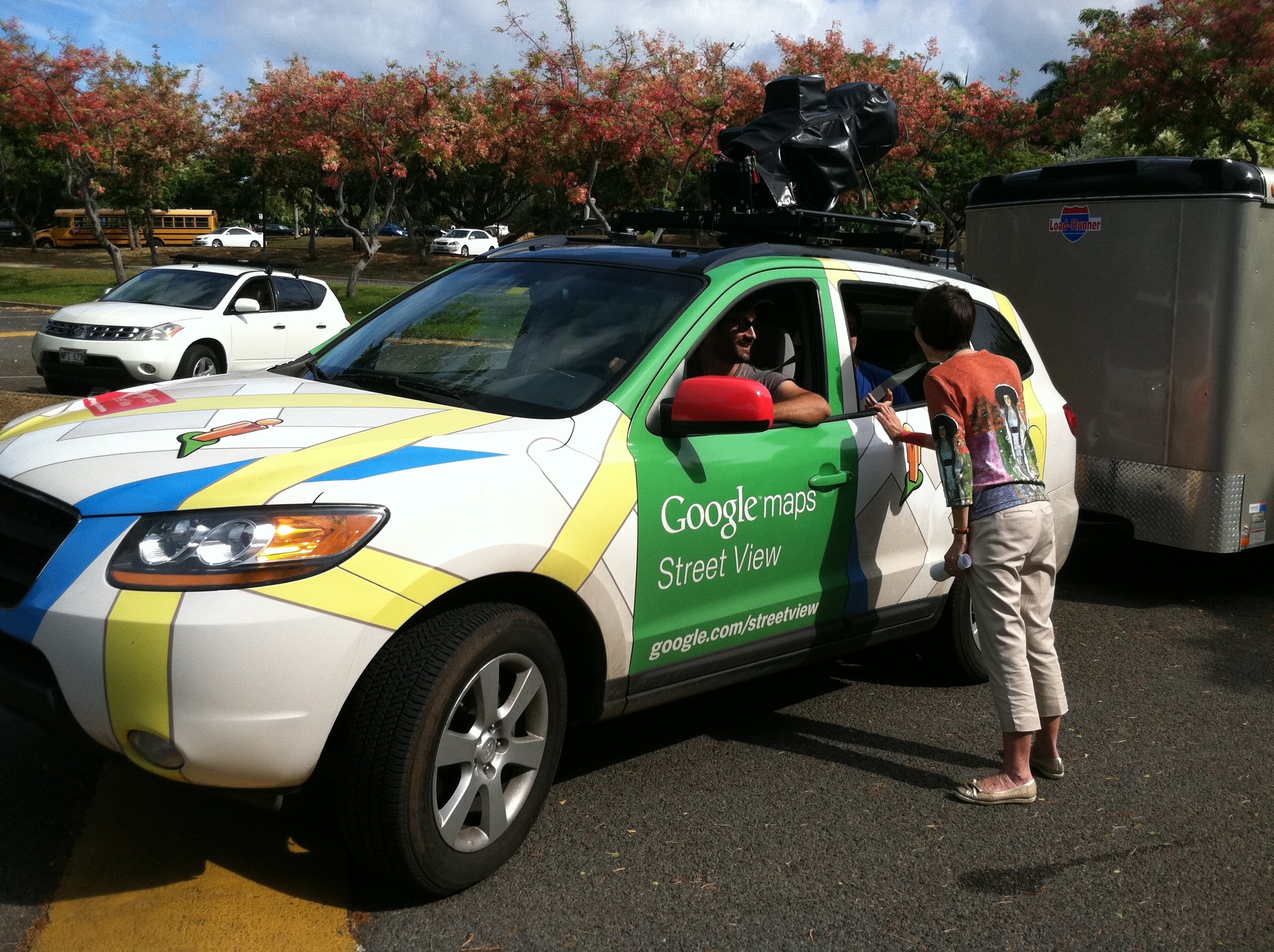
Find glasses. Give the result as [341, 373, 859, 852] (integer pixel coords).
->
[727, 319, 759, 333]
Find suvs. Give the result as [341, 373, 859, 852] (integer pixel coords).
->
[2, 222, 1080, 898]
[32, 264, 352, 395]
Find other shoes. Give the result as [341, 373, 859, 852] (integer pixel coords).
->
[954, 772, 1037, 803]
[1029, 748, 1065, 779]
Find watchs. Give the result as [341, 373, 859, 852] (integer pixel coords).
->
[951, 527, 970, 536]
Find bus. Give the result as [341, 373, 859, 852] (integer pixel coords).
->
[29, 209, 221, 246]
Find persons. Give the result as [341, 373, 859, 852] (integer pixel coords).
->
[869, 283, 1068, 805]
[819, 300, 914, 409]
[685, 296, 833, 429]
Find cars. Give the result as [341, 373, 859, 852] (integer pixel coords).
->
[429, 228, 500, 256]
[319, 224, 354, 237]
[886, 211, 936, 237]
[254, 224, 295, 236]
[482, 223, 509, 236]
[191, 226, 268, 247]
[414, 224, 446, 237]
[376, 222, 409, 237]
[564, 218, 612, 237]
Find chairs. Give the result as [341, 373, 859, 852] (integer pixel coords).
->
[528, 285, 939, 426]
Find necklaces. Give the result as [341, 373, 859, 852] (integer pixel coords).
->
[947, 346, 973, 361]
